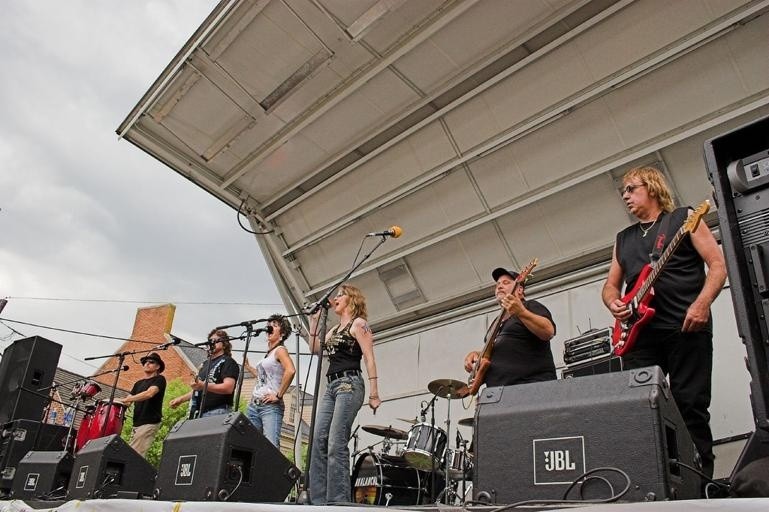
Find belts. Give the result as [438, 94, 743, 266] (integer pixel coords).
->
[328, 371, 358, 383]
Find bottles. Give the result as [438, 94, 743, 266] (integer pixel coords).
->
[63, 406, 76, 427]
[50, 408, 57, 424]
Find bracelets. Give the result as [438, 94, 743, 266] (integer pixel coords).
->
[368, 375, 379, 381]
[275, 391, 285, 400]
[369, 394, 381, 401]
[310, 333, 319, 337]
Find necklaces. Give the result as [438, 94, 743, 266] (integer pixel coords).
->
[637, 210, 663, 239]
[264, 343, 281, 358]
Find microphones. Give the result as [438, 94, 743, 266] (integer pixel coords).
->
[434, 385, 444, 398]
[152, 338, 182, 349]
[368, 225, 401, 238]
[318, 299, 335, 307]
[113, 366, 130, 371]
[351, 424, 360, 436]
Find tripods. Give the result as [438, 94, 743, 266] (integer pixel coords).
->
[435, 419, 463, 505]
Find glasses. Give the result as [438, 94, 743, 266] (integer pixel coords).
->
[337, 291, 346, 296]
[621, 184, 645, 195]
[144, 360, 155, 363]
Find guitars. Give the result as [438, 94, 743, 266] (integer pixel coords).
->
[613, 201, 710, 355]
[189, 374, 202, 419]
[469, 258, 537, 396]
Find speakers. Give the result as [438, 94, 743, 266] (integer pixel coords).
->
[65, 433, 155, 500]
[7, 450, 74, 500]
[0, 335, 63, 423]
[152, 411, 302, 502]
[471, 365, 703, 505]
[1, 419, 41, 492]
[254, 326, 275, 336]
[0, 343, 13, 391]
[703, 115, 769, 484]
[36, 424, 77, 453]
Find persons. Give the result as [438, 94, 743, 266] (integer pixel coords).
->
[600, 164, 728, 483]
[243, 314, 295, 453]
[305, 284, 382, 504]
[168, 329, 239, 419]
[463, 268, 557, 387]
[116, 352, 167, 458]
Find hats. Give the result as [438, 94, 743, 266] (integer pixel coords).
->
[140, 353, 164, 372]
[492, 268, 519, 282]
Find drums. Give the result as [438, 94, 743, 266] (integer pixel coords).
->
[440, 449, 472, 480]
[72, 383, 82, 397]
[79, 380, 101, 400]
[405, 422, 447, 472]
[351, 452, 422, 507]
[75, 404, 96, 453]
[98, 399, 127, 437]
[422, 472, 458, 506]
[86, 401, 106, 442]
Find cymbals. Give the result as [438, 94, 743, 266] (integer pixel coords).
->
[428, 378, 469, 399]
[362, 425, 408, 440]
